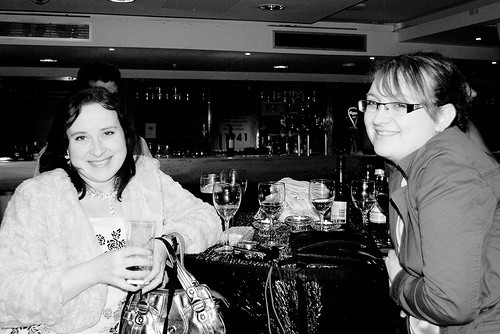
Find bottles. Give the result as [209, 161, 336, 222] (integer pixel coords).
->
[225, 125, 236, 157]
[10, 142, 40, 162]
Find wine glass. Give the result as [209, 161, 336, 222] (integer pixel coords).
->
[220, 167, 249, 226]
[350, 179, 378, 239]
[309, 178, 336, 232]
[257, 181, 286, 248]
[211, 182, 242, 252]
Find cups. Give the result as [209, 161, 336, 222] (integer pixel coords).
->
[199, 172, 227, 204]
[286, 215, 314, 247]
[124, 218, 156, 285]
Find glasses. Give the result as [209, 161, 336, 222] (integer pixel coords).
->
[358, 100, 425, 115]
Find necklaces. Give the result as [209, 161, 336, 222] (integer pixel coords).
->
[83, 178, 123, 217]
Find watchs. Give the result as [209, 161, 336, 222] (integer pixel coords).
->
[162, 232, 179, 256]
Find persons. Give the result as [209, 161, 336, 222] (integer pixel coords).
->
[34, 62, 154, 168]
[358, 50, 500, 333]
[0, 87, 224, 334]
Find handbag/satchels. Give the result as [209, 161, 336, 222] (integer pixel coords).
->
[120, 232, 230, 334]
